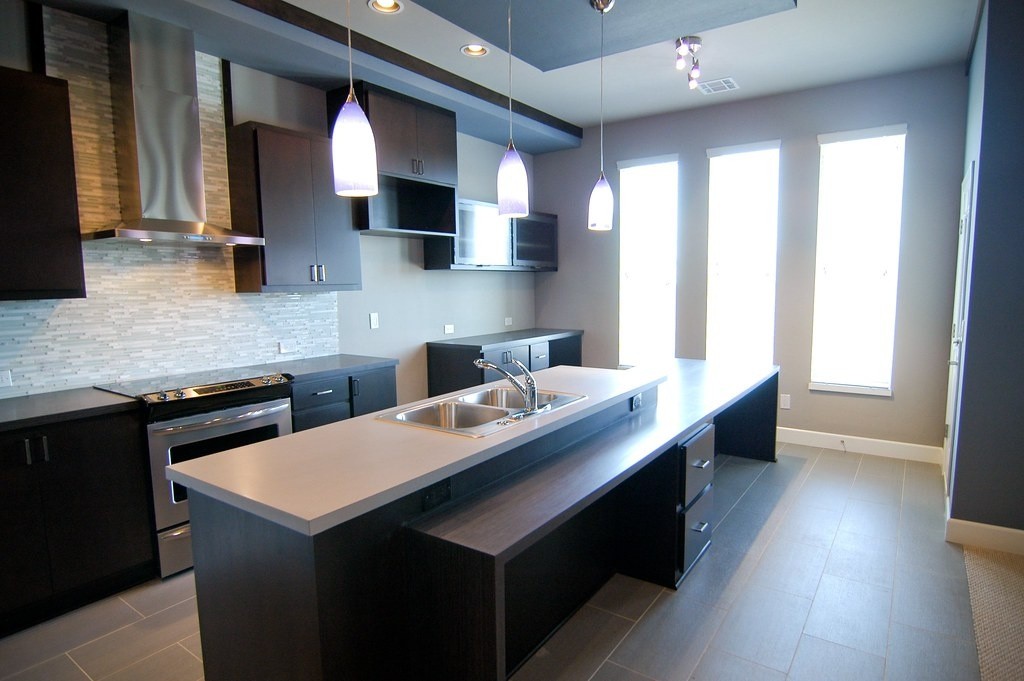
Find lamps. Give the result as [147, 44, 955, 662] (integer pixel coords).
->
[588, 0, 613, 231]
[332, 0, 379, 198]
[497, 1, 529, 218]
[675, 35, 702, 90]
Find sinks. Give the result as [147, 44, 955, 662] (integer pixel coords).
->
[374, 399, 528, 439]
[445, 384, 588, 411]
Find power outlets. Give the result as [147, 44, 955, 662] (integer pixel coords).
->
[444, 325, 454, 334]
[0, 370, 12, 387]
[780, 394, 791, 409]
[279, 341, 297, 353]
[504, 317, 512, 326]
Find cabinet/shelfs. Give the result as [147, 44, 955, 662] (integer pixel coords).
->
[0, 411, 159, 640]
[607, 417, 717, 590]
[423, 197, 559, 272]
[326, 80, 460, 238]
[427, 335, 583, 398]
[291, 366, 398, 432]
[0, 66, 87, 299]
[227, 120, 362, 292]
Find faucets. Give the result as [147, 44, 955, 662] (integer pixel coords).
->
[472, 358, 552, 422]
